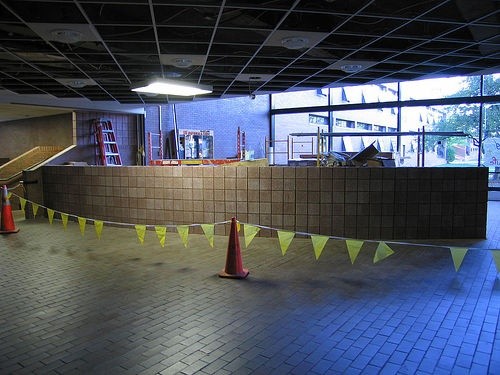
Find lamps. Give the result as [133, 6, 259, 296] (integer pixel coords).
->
[52, 30, 83, 43]
[341, 64, 362, 73]
[280, 36, 309, 50]
[129, 76, 213, 97]
[170, 58, 193, 68]
[66, 80, 87, 88]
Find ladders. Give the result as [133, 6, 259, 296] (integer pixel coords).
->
[92, 117, 122, 168]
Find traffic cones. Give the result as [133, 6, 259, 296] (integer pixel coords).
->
[217, 217, 251, 280]
[1, 184, 21, 234]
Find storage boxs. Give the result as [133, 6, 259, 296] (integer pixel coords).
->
[347, 141, 396, 167]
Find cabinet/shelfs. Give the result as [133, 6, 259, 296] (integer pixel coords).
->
[168, 128, 215, 159]
